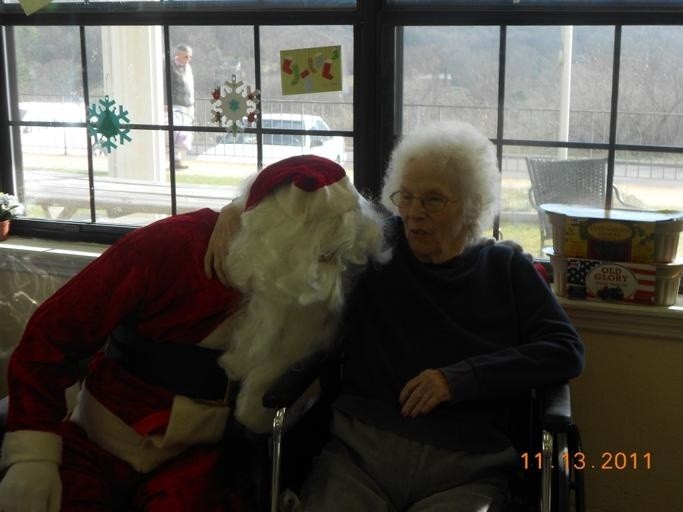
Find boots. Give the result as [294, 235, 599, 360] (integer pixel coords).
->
[175, 160, 188, 169]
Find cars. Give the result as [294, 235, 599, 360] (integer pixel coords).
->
[198, 112, 347, 168]
[18, 101, 99, 145]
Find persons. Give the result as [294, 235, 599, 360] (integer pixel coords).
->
[205, 120, 583, 512]
[164, 44, 195, 170]
[0, 154, 380, 511]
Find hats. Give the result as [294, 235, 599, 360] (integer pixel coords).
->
[240, 154, 359, 227]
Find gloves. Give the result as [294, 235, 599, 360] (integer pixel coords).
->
[0, 461, 62, 511]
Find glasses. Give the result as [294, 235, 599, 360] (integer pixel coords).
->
[389, 190, 459, 211]
[186, 55, 192, 59]
